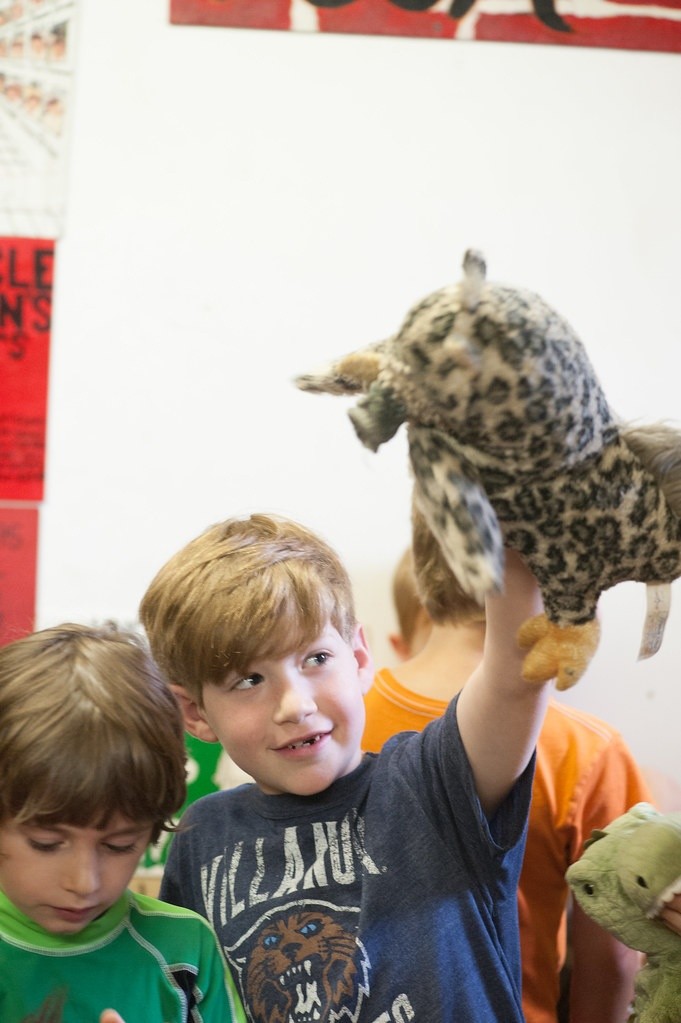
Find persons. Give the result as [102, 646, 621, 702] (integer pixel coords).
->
[0, 623, 246, 1023]
[138, 515, 553, 1023]
[359, 546, 661, 1023]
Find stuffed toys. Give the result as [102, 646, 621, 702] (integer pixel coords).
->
[298, 248, 681, 693]
[564, 799, 681, 1023]
